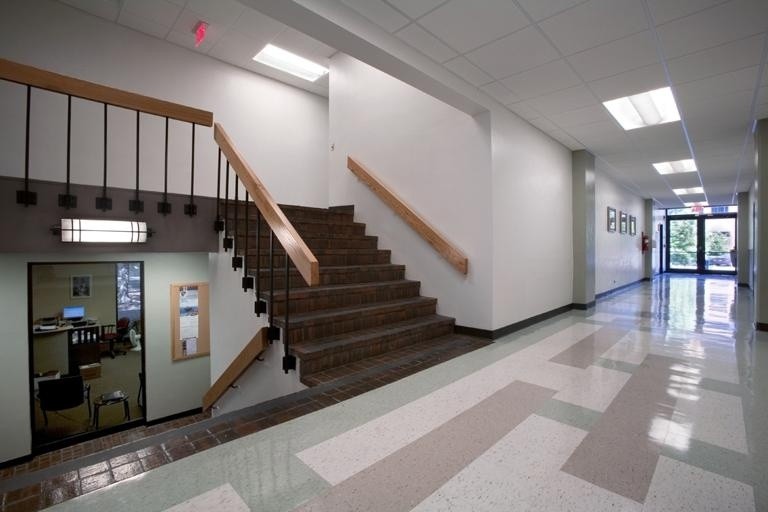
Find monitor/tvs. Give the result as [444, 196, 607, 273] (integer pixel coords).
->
[63, 305, 84, 321]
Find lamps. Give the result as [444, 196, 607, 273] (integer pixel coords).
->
[49, 217, 153, 245]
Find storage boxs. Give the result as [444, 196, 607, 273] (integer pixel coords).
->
[79, 362, 101, 379]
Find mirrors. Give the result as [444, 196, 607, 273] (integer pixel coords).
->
[27, 260, 147, 456]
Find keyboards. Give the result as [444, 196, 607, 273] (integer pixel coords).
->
[71, 321, 87, 327]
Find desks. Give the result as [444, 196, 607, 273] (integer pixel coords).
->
[33, 316, 101, 376]
[93, 391, 131, 429]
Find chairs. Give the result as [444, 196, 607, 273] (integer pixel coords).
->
[34, 374, 92, 426]
[100, 316, 130, 358]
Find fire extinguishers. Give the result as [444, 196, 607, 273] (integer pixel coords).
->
[643, 233, 649, 251]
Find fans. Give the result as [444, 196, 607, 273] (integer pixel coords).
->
[130, 329, 142, 351]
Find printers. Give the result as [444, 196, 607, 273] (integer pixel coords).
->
[39, 316, 60, 330]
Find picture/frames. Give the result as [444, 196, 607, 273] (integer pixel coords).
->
[69, 274, 92, 299]
[629, 214, 636, 236]
[620, 210, 627, 234]
[607, 206, 616, 233]
